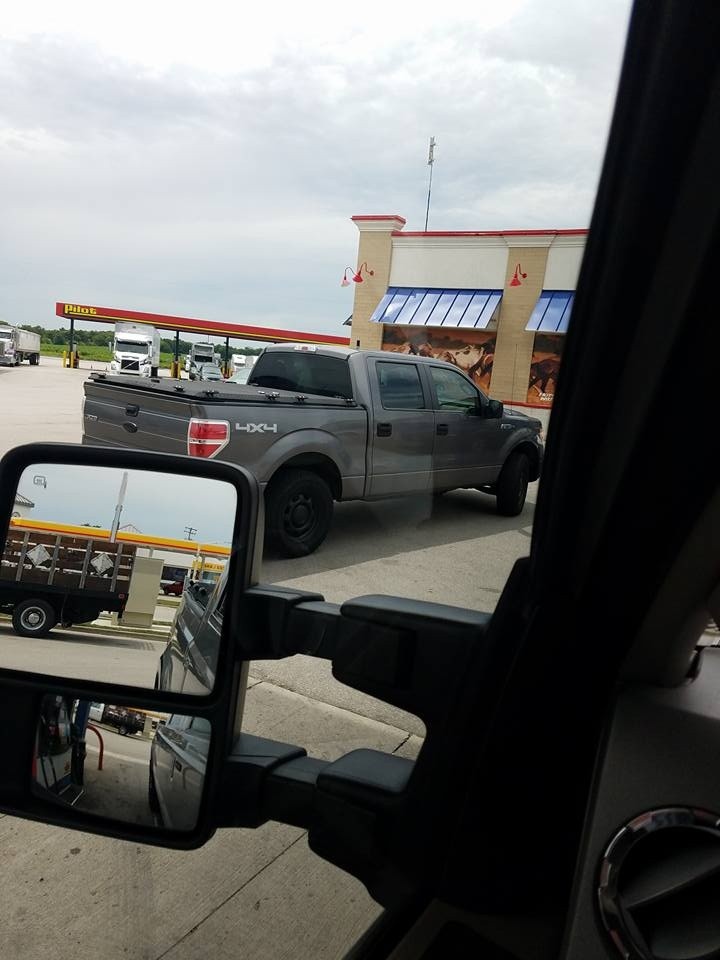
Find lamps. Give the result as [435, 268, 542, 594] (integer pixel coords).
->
[511, 263, 527, 286]
[352, 262, 374, 282]
[340, 267, 355, 287]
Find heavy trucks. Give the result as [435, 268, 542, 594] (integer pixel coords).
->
[184, 344, 221, 380]
[0, 324, 42, 367]
[108, 321, 161, 378]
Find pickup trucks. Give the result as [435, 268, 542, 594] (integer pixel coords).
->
[147, 712, 213, 831]
[79, 344, 547, 560]
[152, 554, 231, 695]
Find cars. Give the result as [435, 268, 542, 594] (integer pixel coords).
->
[195, 365, 224, 382]
[226, 367, 252, 385]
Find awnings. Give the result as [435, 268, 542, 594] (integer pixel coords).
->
[525, 290, 575, 335]
[371, 287, 503, 331]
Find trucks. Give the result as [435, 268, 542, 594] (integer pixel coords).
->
[0, 577, 129, 637]
[76, 699, 147, 738]
